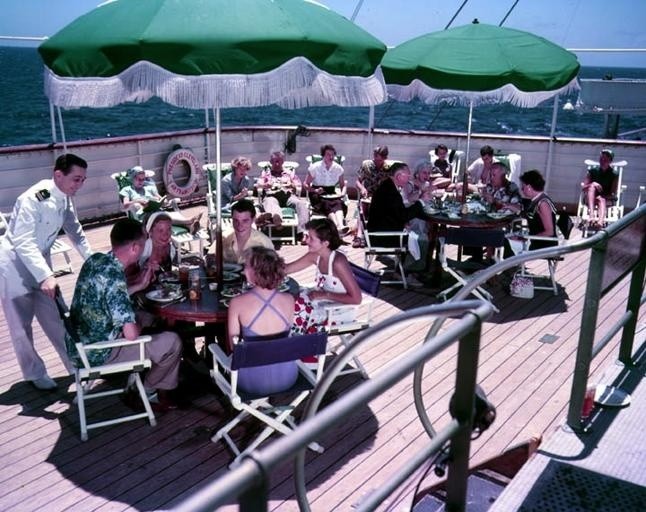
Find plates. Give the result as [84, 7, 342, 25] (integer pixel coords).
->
[319, 194, 343, 201]
[590, 382, 631, 408]
[146, 261, 289, 309]
[427, 194, 515, 223]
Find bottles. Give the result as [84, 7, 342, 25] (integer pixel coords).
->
[252, 178, 257, 196]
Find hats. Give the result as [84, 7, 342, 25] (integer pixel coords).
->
[603, 149, 614, 161]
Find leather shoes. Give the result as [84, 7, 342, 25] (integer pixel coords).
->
[137, 399, 177, 412]
[33, 374, 58, 390]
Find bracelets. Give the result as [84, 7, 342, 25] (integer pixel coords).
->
[325, 294, 331, 300]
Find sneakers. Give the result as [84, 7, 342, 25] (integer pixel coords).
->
[191, 220, 200, 235]
[394, 272, 424, 287]
[256, 213, 366, 248]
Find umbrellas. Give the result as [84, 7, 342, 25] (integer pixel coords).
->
[380, 18, 582, 263]
[38, 0, 387, 292]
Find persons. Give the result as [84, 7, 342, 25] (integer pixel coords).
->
[120, 165, 168, 219]
[136, 208, 182, 268]
[211, 247, 298, 440]
[494, 170, 558, 262]
[302, 145, 349, 233]
[467, 146, 523, 259]
[283, 217, 364, 369]
[581, 148, 619, 227]
[221, 157, 252, 212]
[355, 146, 392, 247]
[432, 146, 453, 180]
[65, 217, 181, 410]
[256, 151, 310, 239]
[1, 153, 93, 391]
[209, 200, 275, 264]
[368, 159, 436, 275]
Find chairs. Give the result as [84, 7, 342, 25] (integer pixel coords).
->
[426, 225, 506, 309]
[52, 285, 153, 437]
[106, 164, 199, 238]
[205, 163, 269, 241]
[427, 150, 463, 182]
[355, 202, 412, 292]
[577, 159, 628, 238]
[255, 159, 304, 247]
[487, 149, 526, 181]
[322, 257, 381, 382]
[357, 154, 402, 201]
[517, 208, 572, 302]
[305, 153, 353, 241]
[2, 210, 73, 275]
[207, 333, 331, 463]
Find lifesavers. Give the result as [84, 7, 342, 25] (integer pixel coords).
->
[163, 148, 200, 197]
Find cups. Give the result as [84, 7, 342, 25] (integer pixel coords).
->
[581, 384, 595, 418]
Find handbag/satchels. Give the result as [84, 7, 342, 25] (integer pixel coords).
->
[509, 269, 535, 299]
